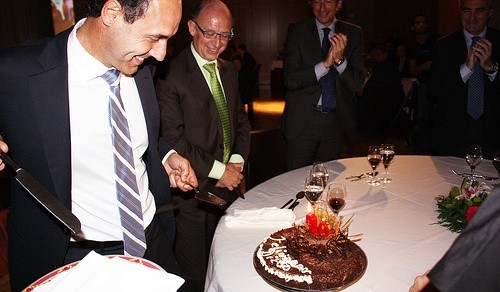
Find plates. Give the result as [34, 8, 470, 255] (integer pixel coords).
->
[21, 255, 176, 292]
[253, 235, 367, 292]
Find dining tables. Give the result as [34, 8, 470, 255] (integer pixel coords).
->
[204, 155, 498, 292]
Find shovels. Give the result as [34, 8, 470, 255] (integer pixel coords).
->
[183, 181, 227, 206]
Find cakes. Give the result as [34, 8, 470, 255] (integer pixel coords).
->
[255, 222, 364, 291]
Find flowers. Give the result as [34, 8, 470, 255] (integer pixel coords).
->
[430, 177, 491, 234]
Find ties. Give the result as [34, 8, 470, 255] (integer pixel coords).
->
[466, 36, 485, 119]
[100, 69, 146, 259]
[321, 28, 338, 112]
[203, 63, 231, 163]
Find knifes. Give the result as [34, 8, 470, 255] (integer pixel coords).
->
[184, 180, 228, 205]
[0, 147, 82, 234]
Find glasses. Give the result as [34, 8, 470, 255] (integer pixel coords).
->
[193, 20, 234, 41]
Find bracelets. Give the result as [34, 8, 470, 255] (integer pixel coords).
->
[334, 57, 345, 66]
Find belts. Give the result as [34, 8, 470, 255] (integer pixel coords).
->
[70, 241, 123, 249]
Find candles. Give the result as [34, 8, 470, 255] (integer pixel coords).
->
[305, 202, 329, 238]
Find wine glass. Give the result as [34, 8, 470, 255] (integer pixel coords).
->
[379, 144, 395, 183]
[366, 146, 382, 185]
[326, 183, 348, 219]
[492, 152, 500, 177]
[310, 162, 329, 207]
[465, 144, 484, 179]
[305, 174, 324, 211]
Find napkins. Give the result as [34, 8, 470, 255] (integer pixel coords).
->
[49, 250, 186, 292]
[223, 207, 293, 225]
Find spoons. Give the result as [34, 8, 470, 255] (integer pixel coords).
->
[289, 190, 306, 210]
[353, 171, 377, 182]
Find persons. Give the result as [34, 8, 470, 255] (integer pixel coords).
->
[154, 0, 251, 292]
[285, 0, 368, 169]
[0, 0, 197, 292]
[0, 135, 9, 171]
[409, 185, 500, 292]
[426, 0, 500, 159]
[234, 14, 442, 129]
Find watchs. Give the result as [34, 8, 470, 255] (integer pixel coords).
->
[486, 64, 498, 73]
